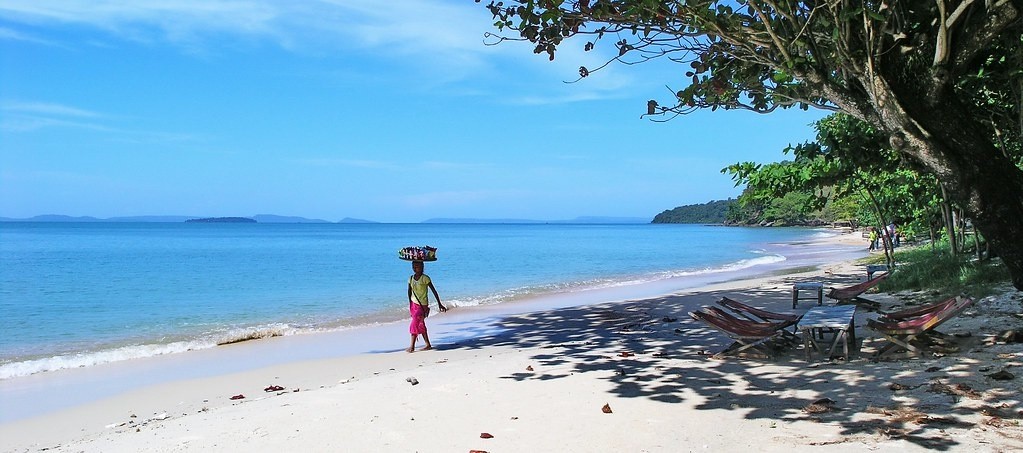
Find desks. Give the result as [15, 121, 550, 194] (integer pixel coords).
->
[797, 305, 856, 361]
[792, 283, 824, 308]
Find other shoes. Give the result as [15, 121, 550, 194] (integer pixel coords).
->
[423, 347, 431, 350]
[406, 349, 414, 353]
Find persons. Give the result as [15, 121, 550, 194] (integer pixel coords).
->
[405, 259, 447, 352]
[867, 222, 900, 253]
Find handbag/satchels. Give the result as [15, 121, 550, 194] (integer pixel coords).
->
[425, 307, 429, 317]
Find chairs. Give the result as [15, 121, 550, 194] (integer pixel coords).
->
[862, 293, 974, 360]
[825, 271, 890, 308]
[716, 296, 800, 350]
[687, 305, 798, 360]
[876, 292, 968, 342]
